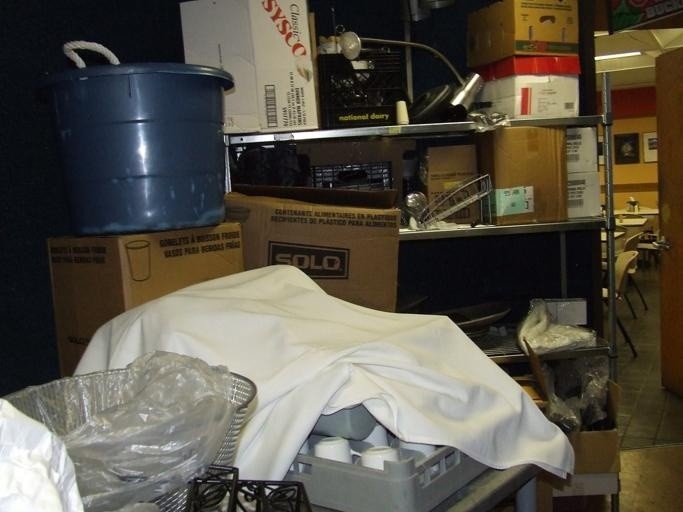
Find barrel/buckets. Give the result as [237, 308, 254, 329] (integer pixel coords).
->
[50, 38, 235, 236]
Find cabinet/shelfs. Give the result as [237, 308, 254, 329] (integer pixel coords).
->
[226, 68, 607, 366]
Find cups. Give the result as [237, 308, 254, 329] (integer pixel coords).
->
[399, 439, 438, 472]
[364, 424, 389, 446]
[355, 446, 398, 471]
[314, 436, 362, 464]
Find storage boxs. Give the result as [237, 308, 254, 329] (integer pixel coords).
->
[465, 0, 580, 67]
[562, 126, 597, 173]
[179, 1, 320, 134]
[523, 335, 622, 473]
[477, 119, 564, 223]
[224, 191, 402, 316]
[424, 130, 483, 224]
[567, 175, 598, 218]
[45, 221, 243, 378]
[474, 55, 583, 118]
[529, 297, 587, 327]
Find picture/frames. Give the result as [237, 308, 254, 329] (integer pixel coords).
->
[642, 130, 660, 163]
[597, 134, 605, 166]
[614, 133, 639, 165]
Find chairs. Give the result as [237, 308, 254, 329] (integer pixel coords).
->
[624, 231, 648, 320]
[602, 250, 648, 358]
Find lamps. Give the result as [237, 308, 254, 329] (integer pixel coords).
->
[594, 51, 642, 62]
[336, 28, 485, 120]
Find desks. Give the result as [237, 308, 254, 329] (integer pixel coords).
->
[612, 218, 648, 226]
[614, 208, 660, 215]
[601, 229, 626, 243]
